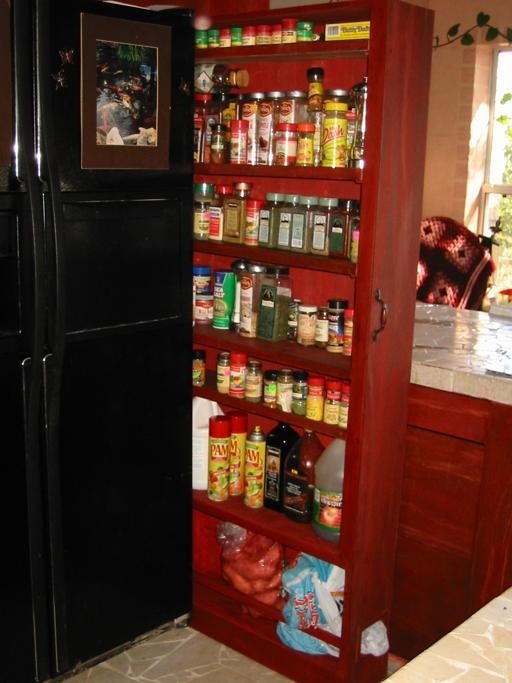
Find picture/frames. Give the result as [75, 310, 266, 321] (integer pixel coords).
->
[80, 13, 176, 173]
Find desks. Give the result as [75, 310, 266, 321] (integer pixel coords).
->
[392, 291, 511, 661]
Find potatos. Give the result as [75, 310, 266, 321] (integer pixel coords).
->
[222, 539, 287, 618]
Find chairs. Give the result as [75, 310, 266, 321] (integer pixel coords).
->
[414, 212, 492, 315]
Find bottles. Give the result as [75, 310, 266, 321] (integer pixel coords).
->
[191, 255, 352, 358]
[245, 423, 265, 508]
[191, 349, 350, 429]
[191, 64, 367, 166]
[283, 426, 326, 521]
[193, 179, 360, 263]
[209, 411, 248, 502]
[264, 419, 301, 511]
[310, 434, 346, 544]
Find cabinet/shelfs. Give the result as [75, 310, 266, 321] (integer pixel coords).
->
[190, 5, 440, 681]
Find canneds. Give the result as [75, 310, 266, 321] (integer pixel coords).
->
[263, 369, 308, 415]
[296, 305, 318, 346]
[195, 295, 214, 326]
[271, 124, 298, 167]
